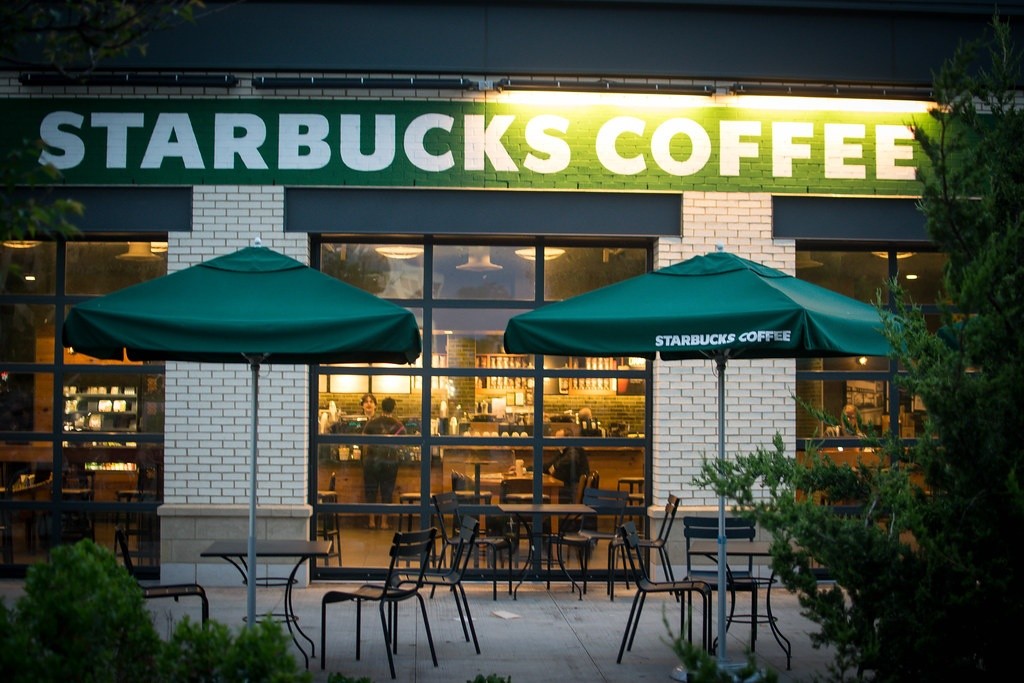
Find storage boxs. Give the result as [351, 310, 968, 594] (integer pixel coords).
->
[881, 404, 927, 439]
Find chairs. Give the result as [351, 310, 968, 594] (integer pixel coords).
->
[321, 526, 437, 680]
[616, 520, 714, 664]
[388, 515, 481, 655]
[114, 525, 211, 630]
[1, 462, 161, 566]
[309, 471, 681, 602]
[681, 517, 757, 654]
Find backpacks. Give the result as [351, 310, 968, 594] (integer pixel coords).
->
[370, 416, 406, 466]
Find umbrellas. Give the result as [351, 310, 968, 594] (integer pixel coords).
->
[55, 235, 422, 631]
[502, 243, 952, 683]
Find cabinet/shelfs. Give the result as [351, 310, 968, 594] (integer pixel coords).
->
[441, 444, 644, 532]
[474, 337, 618, 398]
[64, 369, 140, 432]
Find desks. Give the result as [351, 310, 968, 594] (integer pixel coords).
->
[494, 502, 597, 602]
[688, 540, 811, 670]
[200, 541, 333, 669]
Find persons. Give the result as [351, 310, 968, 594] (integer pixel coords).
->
[823, 404, 866, 438]
[508, 427, 590, 534]
[361, 393, 381, 427]
[361, 397, 406, 527]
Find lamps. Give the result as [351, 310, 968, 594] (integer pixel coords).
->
[374, 244, 424, 260]
[514, 247, 566, 261]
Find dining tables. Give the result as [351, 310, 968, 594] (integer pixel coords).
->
[0, 444, 68, 468]
[468, 471, 565, 562]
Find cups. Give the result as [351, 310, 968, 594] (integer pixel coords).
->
[440, 401, 447, 418]
[432, 420, 437, 434]
[328, 400, 337, 422]
[481, 403, 488, 414]
[464, 431, 529, 437]
[450, 417, 457, 435]
[516, 459, 524, 476]
[320, 413, 327, 433]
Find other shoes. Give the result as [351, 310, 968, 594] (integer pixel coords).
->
[380, 524, 389, 530]
[369, 523, 375, 529]
